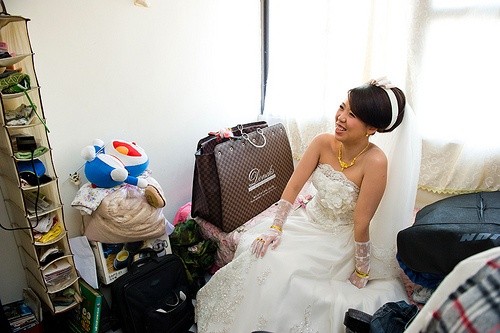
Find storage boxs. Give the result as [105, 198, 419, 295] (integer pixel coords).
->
[90, 232, 173, 284]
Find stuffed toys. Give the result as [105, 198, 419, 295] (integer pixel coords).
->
[83, 139, 150, 187]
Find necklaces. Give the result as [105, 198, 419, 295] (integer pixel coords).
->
[337, 143, 369, 171]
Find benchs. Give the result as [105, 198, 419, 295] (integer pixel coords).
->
[174, 202, 424, 310]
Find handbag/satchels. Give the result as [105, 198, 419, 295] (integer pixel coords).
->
[190, 121, 295, 233]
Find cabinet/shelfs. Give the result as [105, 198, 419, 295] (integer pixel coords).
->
[0, 0, 83, 333]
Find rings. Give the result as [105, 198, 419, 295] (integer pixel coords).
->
[260, 238, 263, 241]
[257, 238, 259, 240]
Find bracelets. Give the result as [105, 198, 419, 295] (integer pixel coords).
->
[271, 225, 282, 231]
[354, 268, 368, 277]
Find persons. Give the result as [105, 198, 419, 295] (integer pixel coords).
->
[195, 79, 410, 333]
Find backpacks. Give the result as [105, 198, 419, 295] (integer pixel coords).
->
[108, 247, 198, 333]
[169, 219, 217, 285]
